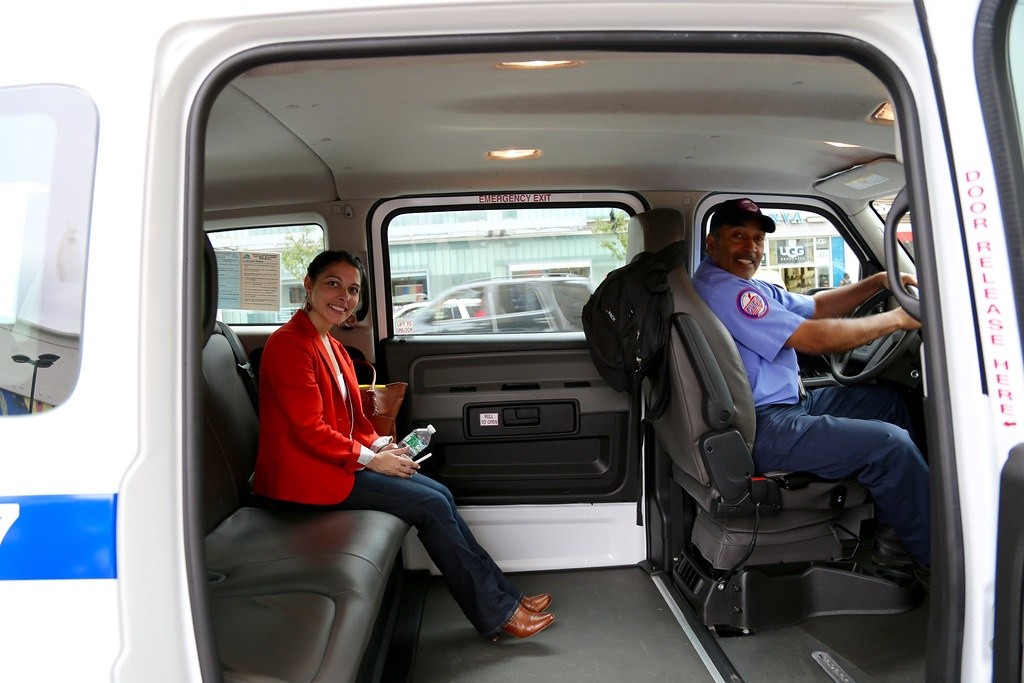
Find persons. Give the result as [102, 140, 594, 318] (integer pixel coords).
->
[839, 273, 852, 287]
[692, 197, 934, 591]
[416, 293, 427, 303]
[254, 250, 555, 642]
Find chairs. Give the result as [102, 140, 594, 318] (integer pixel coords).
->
[627, 206, 875, 636]
[482, 287, 538, 329]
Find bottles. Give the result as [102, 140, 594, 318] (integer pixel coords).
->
[395, 424, 436, 460]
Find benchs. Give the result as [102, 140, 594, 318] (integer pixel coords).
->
[195, 227, 431, 683]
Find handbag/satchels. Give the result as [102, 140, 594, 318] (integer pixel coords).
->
[354, 360, 408, 443]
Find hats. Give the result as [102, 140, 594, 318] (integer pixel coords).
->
[710, 198, 776, 235]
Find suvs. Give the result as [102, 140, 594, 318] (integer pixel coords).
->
[396, 273, 601, 334]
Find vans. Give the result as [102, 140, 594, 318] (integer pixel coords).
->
[0, 1, 1023, 683]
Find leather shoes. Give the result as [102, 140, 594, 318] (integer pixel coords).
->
[870, 518, 920, 572]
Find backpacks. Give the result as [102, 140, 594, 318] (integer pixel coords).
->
[580, 238, 690, 428]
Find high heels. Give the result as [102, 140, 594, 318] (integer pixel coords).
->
[520, 594, 552, 613]
[492, 603, 555, 642]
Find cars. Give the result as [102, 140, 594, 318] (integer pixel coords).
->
[393, 298, 509, 340]
